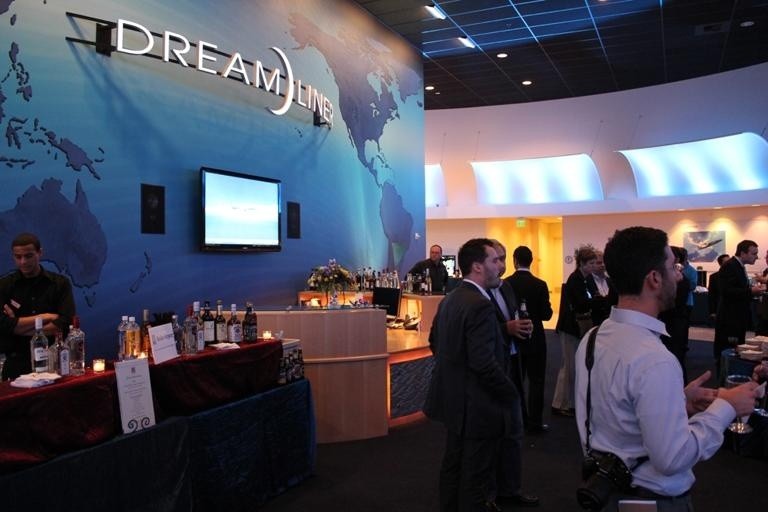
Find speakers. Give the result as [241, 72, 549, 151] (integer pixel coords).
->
[140, 183, 167, 234]
[287, 202, 301, 238]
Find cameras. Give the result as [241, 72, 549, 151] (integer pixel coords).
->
[577, 444, 632, 509]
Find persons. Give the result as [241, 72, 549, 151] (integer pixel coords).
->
[747, 356, 768, 383]
[716, 239, 766, 378]
[1, 233, 80, 385]
[574, 225, 760, 511]
[754, 249, 768, 294]
[486, 238, 542, 505]
[403, 245, 447, 292]
[678, 246, 697, 353]
[707, 254, 730, 366]
[585, 250, 617, 327]
[503, 245, 552, 435]
[657, 246, 689, 366]
[422, 237, 518, 512]
[549, 244, 597, 418]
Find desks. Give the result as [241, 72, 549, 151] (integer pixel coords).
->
[721, 347, 768, 452]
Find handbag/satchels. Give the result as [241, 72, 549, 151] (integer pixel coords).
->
[576, 312, 593, 336]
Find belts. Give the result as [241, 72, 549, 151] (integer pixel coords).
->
[619, 487, 691, 501]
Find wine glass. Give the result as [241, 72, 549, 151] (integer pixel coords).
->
[728, 337, 737, 357]
[724, 374, 753, 435]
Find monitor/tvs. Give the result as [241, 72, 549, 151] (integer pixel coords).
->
[199, 166, 283, 251]
[372, 287, 402, 319]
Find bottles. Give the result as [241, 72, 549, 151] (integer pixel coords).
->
[117, 316, 129, 359]
[277, 347, 305, 385]
[213, 300, 227, 342]
[29, 316, 50, 373]
[518, 298, 531, 338]
[125, 316, 141, 357]
[244, 303, 257, 341]
[202, 300, 215, 344]
[141, 300, 204, 356]
[51, 332, 69, 378]
[69, 317, 85, 376]
[352, 265, 433, 297]
[227, 302, 244, 343]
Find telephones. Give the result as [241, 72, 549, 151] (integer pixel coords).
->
[403, 316, 419, 330]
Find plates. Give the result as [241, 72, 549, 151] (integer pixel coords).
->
[737, 334, 767, 361]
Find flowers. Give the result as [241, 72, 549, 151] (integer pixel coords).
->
[308, 258, 358, 293]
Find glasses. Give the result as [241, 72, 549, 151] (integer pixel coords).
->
[661, 264, 684, 274]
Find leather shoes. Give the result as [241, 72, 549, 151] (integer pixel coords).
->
[529, 424, 548, 432]
[552, 407, 574, 418]
[482, 491, 539, 510]
